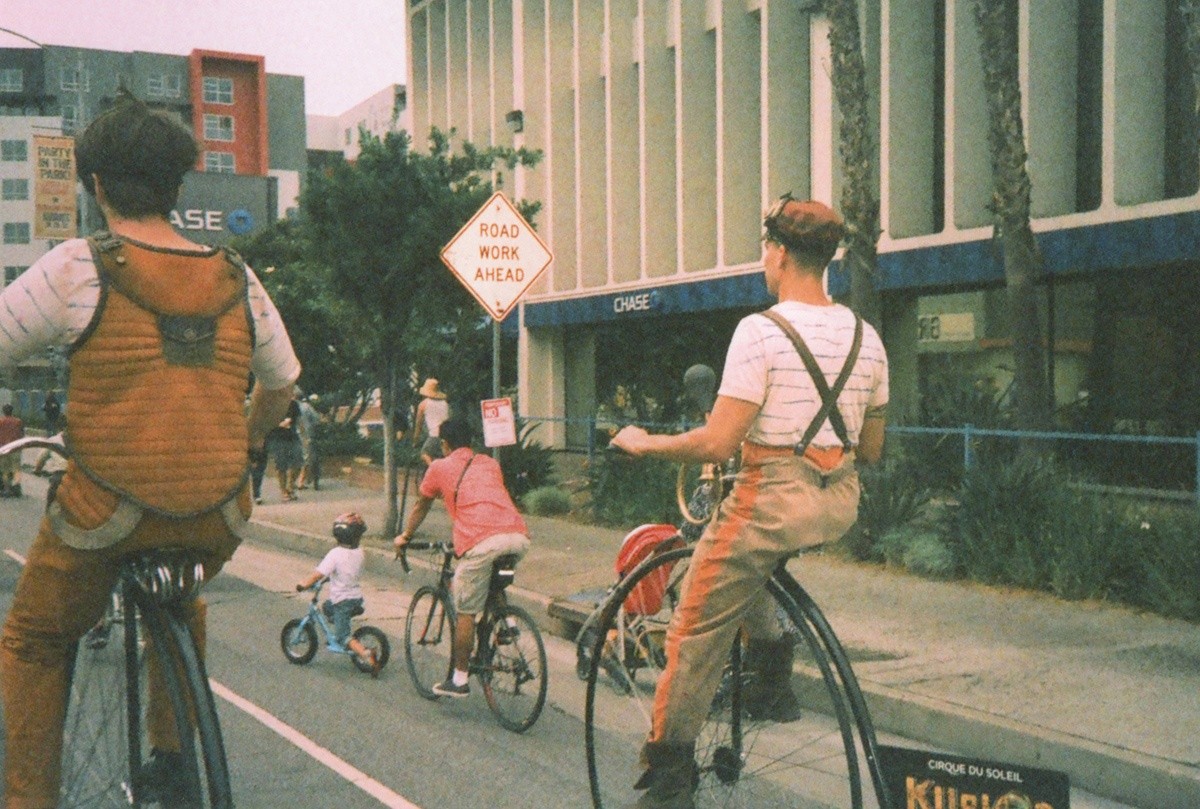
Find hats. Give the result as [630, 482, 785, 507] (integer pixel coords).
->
[418, 376, 448, 400]
[757, 190, 845, 261]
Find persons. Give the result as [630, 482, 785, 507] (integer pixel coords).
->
[0, 404, 25, 495]
[298, 511, 381, 681]
[0, 104, 303, 809]
[411, 378, 450, 468]
[288, 387, 320, 490]
[393, 416, 530, 700]
[738, 586, 802, 723]
[268, 400, 305, 502]
[250, 438, 269, 506]
[41, 390, 61, 438]
[34, 412, 69, 514]
[605, 192, 890, 809]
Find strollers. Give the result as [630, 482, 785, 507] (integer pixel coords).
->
[576, 475, 769, 715]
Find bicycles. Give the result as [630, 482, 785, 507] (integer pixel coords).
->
[392, 542, 560, 729]
[574, 441, 896, 809]
[280, 576, 387, 672]
[0, 436, 238, 809]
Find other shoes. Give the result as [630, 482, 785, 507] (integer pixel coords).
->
[255, 496, 263, 505]
[363, 646, 379, 678]
[431, 680, 471, 698]
[280, 489, 298, 502]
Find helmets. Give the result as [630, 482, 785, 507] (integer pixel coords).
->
[332, 511, 367, 539]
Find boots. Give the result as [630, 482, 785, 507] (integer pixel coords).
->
[718, 631, 805, 724]
[633, 738, 700, 809]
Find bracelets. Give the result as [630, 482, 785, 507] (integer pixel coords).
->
[401, 535, 412, 541]
